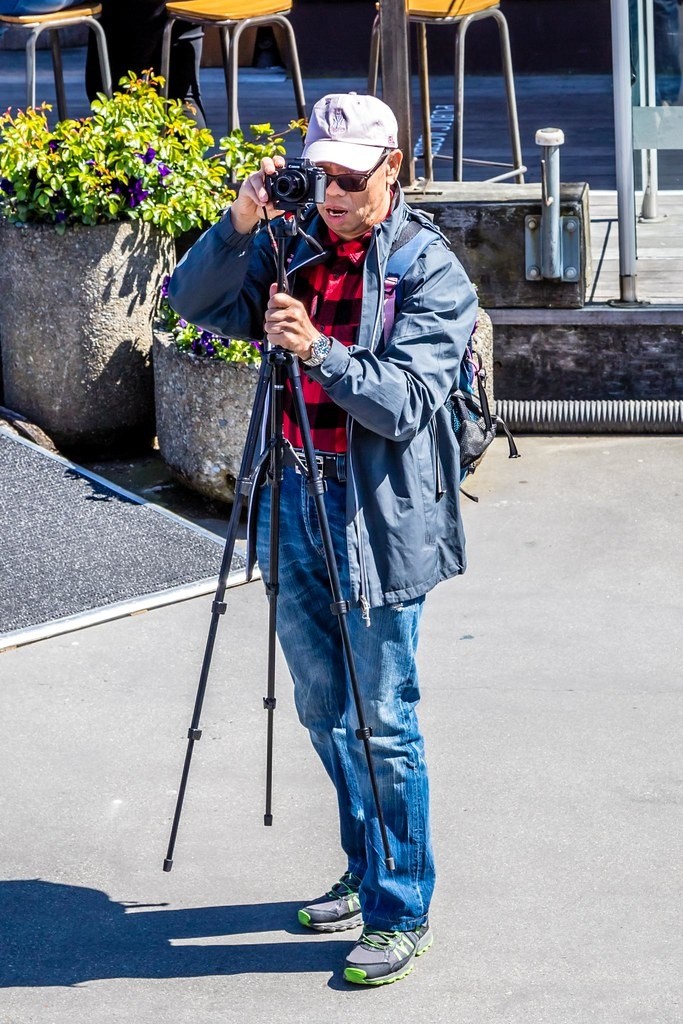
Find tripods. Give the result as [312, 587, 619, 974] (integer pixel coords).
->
[164, 201, 400, 872]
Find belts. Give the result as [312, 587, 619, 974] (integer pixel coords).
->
[279, 450, 348, 478]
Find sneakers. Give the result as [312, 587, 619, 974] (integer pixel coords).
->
[298, 871, 364, 933]
[343, 918, 433, 986]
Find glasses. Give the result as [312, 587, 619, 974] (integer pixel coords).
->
[325, 147, 392, 192]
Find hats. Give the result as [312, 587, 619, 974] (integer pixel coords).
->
[300, 92, 398, 171]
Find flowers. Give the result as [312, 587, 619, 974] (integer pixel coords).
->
[0, 71, 307, 368]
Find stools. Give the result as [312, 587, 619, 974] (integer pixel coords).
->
[158, 0, 308, 152]
[373, 0, 526, 183]
[0, 3, 113, 118]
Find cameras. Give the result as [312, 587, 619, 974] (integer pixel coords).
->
[264, 157, 327, 210]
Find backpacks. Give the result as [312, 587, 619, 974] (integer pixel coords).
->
[284, 204, 497, 475]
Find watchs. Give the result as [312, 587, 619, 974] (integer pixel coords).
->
[304, 335, 331, 367]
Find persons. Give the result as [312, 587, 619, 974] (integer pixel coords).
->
[167, 89, 480, 986]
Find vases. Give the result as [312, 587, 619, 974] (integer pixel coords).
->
[0, 220, 176, 464]
[153, 330, 265, 505]
[468, 306, 493, 473]
